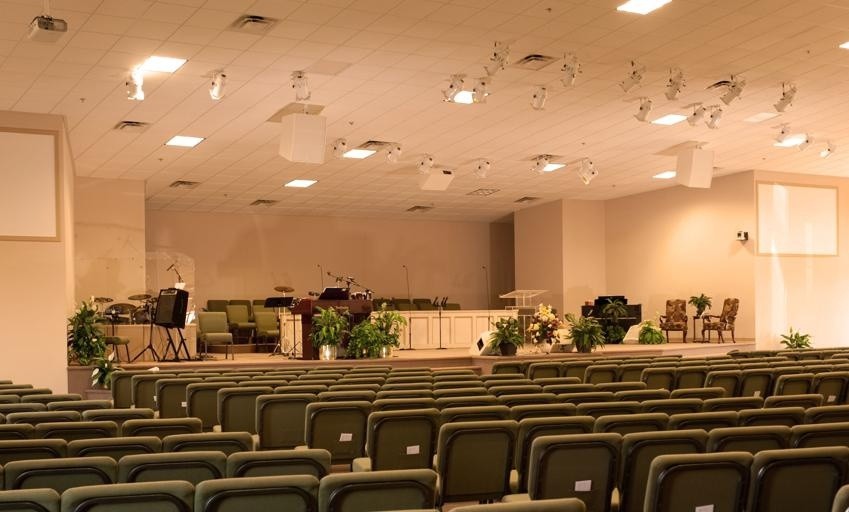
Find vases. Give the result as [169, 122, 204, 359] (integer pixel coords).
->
[542, 340, 551, 355]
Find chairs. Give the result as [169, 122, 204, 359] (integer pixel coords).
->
[0, 380, 112, 512]
[496, 349, 848, 512]
[701, 298, 739, 343]
[199, 299, 288, 361]
[112, 367, 495, 512]
[659, 299, 688, 343]
[98, 319, 130, 363]
[373, 297, 460, 312]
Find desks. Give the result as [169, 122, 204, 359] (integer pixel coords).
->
[103, 322, 198, 360]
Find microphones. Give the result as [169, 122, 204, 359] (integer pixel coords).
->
[482, 266, 500, 310]
[167, 264, 173, 272]
[317, 265, 327, 299]
[308, 291, 320, 296]
[401, 265, 421, 311]
[327, 271, 347, 289]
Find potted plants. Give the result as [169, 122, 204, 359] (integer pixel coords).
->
[602, 299, 627, 345]
[310, 303, 408, 359]
[487, 317, 524, 356]
[688, 294, 711, 315]
[84, 351, 118, 400]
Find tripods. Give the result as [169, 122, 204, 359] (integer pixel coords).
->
[268, 304, 294, 356]
[130, 306, 160, 363]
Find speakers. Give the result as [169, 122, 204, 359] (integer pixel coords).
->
[278, 113, 327, 164]
[154, 288, 189, 329]
[546, 329, 574, 353]
[675, 147, 714, 189]
[475, 332, 500, 356]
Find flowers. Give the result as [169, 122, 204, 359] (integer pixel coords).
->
[68, 295, 109, 395]
[525, 303, 563, 345]
[564, 313, 606, 352]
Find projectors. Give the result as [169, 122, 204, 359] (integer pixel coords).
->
[26, 14, 68, 43]
[419, 169, 453, 191]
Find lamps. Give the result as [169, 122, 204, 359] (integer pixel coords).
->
[387, 146, 401, 162]
[476, 161, 491, 179]
[532, 86, 547, 111]
[665, 68, 684, 103]
[707, 107, 723, 130]
[289, 69, 310, 101]
[126, 74, 144, 102]
[618, 64, 644, 93]
[579, 160, 598, 184]
[442, 79, 464, 101]
[208, 72, 228, 101]
[561, 57, 579, 88]
[772, 87, 797, 115]
[415, 155, 434, 175]
[528, 154, 548, 177]
[777, 123, 791, 143]
[799, 136, 817, 152]
[485, 49, 509, 78]
[474, 82, 489, 104]
[331, 138, 347, 161]
[633, 100, 653, 124]
[688, 104, 706, 130]
[719, 78, 746, 105]
[821, 144, 836, 159]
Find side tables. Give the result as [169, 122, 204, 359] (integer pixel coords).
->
[693, 315, 711, 343]
